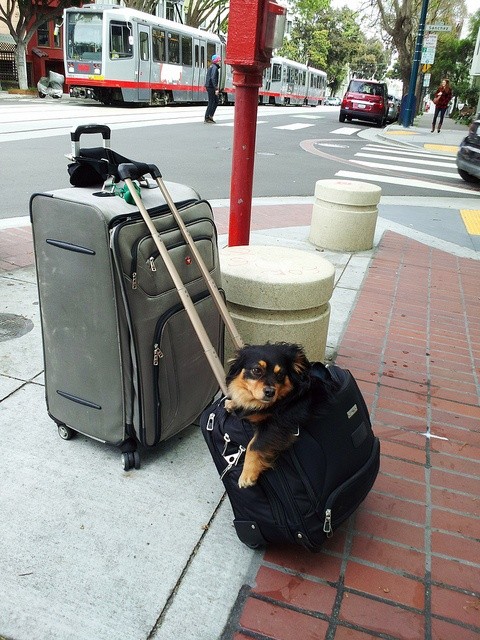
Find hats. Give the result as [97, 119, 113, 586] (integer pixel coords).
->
[212, 54, 221, 63]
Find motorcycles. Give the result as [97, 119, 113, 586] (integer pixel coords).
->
[37, 70, 65, 99]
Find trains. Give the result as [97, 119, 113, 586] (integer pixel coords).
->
[53, 3, 327, 108]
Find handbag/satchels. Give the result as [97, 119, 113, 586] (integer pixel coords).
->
[433, 96, 441, 103]
[67, 147, 150, 188]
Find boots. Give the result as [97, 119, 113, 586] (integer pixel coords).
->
[431, 123, 435, 132]
[437, 123, 442, 133]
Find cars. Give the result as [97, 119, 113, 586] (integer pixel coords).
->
[387, 95, 397, 123]
[455, 112, 480, 187]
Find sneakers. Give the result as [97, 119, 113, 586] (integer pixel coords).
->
[204, 117, 217, 124]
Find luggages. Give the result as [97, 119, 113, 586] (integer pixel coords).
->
[29, 124, 226, 470]
[117, 163, 380, 554]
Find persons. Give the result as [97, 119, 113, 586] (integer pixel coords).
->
[431, 79, 452, 133]
[203, 55, 221, 124]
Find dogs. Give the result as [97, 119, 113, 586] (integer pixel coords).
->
[223, 340, 339, 490]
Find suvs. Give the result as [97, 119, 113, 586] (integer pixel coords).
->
[339, 80, 388, 128]
